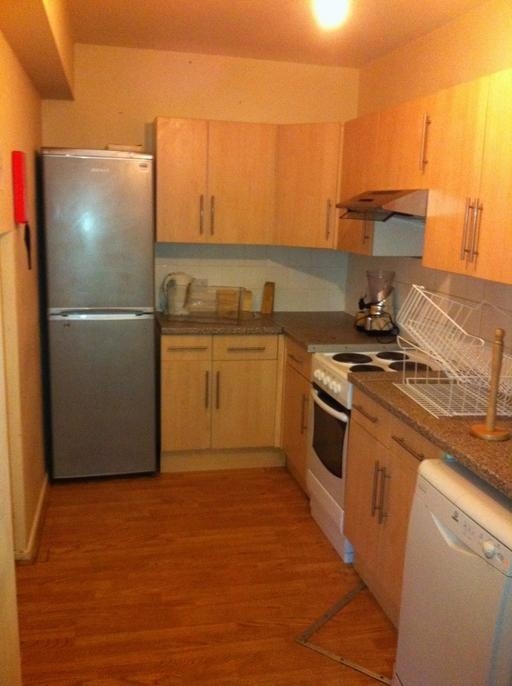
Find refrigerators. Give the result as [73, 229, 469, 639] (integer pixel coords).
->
[34, 143, 161, 484]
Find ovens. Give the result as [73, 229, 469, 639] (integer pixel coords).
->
[306, 380, 351, 514]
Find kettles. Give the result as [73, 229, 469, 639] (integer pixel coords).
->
[160, 271, 196, 316]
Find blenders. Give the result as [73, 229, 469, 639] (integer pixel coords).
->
[354, 268, 398, 337]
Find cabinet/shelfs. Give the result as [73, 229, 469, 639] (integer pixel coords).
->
[154, 117, 277, 245]
[342, 385, 441, 633]
[277, 123, 375, 256]
[283, 336, 313, 500]
[337, 112, 424, 257]
[420, 66, 512, 285]
[160, 334, 284, 471]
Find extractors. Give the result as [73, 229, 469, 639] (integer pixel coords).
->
[333, 184, 429, 229]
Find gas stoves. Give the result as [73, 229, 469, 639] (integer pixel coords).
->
[313, 346, 444, 381]
